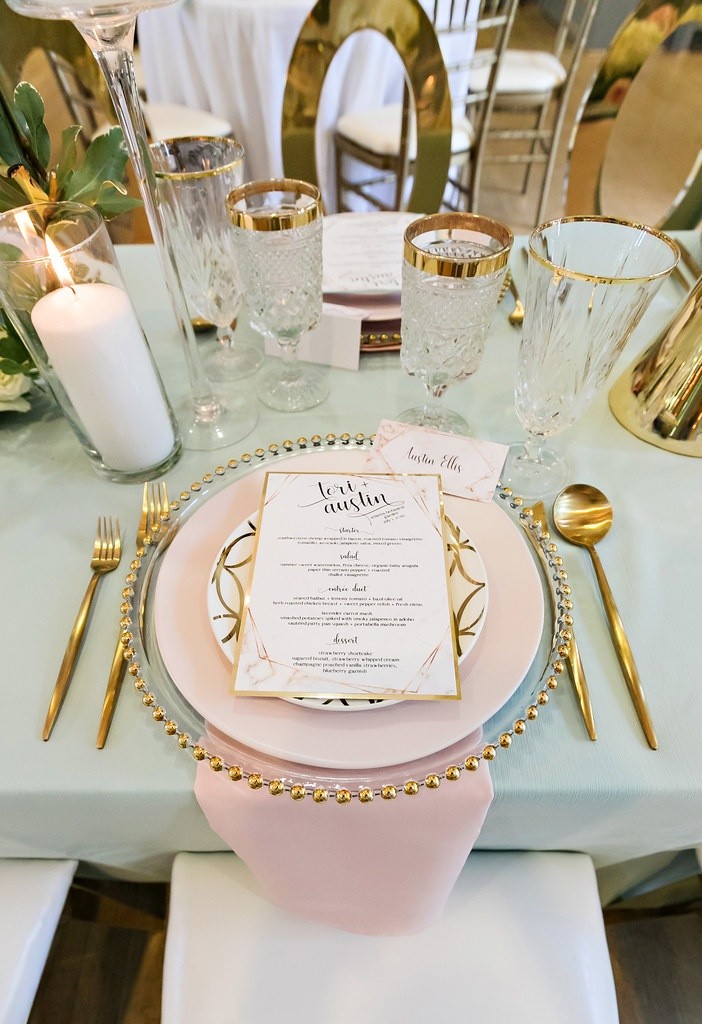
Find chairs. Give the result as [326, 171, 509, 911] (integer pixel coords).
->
[0, 853, 621, 1024]
[41, 0, 702, 231]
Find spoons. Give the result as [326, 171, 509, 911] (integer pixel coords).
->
[552, 484, 659, 751]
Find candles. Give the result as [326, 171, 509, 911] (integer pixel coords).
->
[30, 233, 175, 472]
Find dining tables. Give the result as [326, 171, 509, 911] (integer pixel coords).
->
[0, 235, 702, 858]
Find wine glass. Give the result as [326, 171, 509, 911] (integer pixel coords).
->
[149, 134, 264, 380]
[225, 177, 332, 412]
[5, 0, 260, 450]
[394, 211, 514, 440]
[498, 214, 680, 500]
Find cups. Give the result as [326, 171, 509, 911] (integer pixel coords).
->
[0, 200, 184, 483]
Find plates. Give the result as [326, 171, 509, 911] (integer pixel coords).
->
[207, 506, 488, 713]
[313, 208, 425, 352]
[121, 434, 575, 805]
[156, 450, 544, 771]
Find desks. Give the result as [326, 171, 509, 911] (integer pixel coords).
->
[136, 0, 482, 213]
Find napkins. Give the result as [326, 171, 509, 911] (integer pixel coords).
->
[194, 722, 495, 934]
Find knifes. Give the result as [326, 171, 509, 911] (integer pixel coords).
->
[532, 501, 598, 741]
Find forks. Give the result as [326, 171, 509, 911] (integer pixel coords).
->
[508, 279, 524, 327]
[97, 481, 168, 750]
[42, 517, 120, 741]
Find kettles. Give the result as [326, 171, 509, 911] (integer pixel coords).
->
[607, 273, 702, 459]
